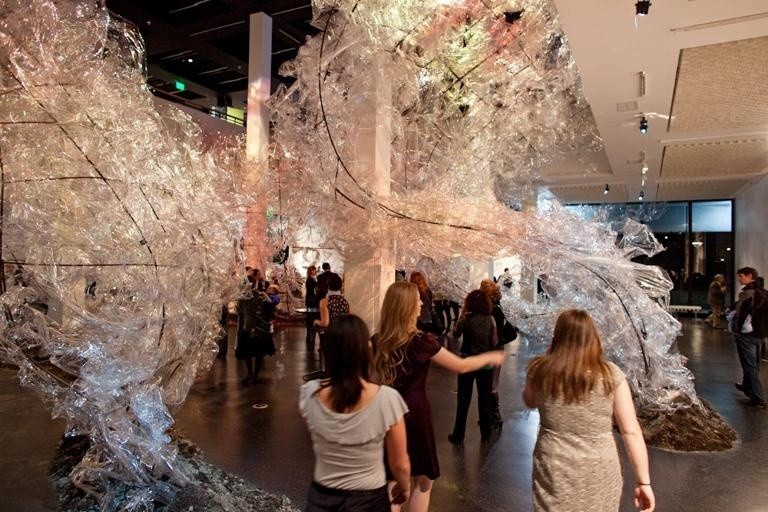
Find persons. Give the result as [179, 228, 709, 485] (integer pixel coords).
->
[524, 307, 655, 512]
[729, 267, 768, 409]
[239, 266, 281, 384]
[703, 273, 728, 329]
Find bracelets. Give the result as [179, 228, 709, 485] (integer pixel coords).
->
[636, 481, 650, 486]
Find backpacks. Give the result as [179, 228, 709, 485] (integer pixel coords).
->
[753, 286, 768, 338]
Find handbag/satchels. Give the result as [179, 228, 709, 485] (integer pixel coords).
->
[492, 304, 518, 344]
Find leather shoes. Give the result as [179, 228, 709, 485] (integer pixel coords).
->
[741, 399, 767, 409]
[735, 382, 743, 392]
